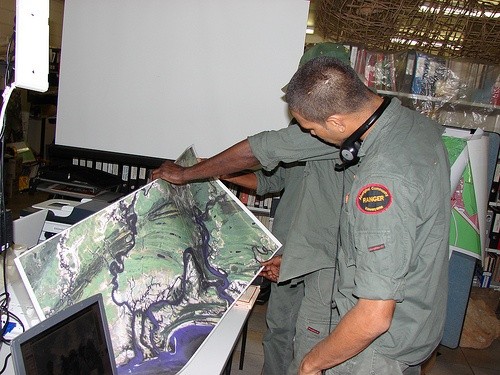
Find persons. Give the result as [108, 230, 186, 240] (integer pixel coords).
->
[151, 56, 344, 375]
[283, 55, 452, 373]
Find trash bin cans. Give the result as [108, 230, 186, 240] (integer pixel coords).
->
[4, 141, 36, 194]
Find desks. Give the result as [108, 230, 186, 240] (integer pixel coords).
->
[178, 281, 262, 375]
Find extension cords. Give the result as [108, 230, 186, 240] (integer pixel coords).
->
[1, 285, 28, 341]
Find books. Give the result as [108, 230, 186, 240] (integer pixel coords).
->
[342, 43, 441, 98]
[471, 157, 500, 289]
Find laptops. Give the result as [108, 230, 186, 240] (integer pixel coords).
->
[10, 293, 118, 375]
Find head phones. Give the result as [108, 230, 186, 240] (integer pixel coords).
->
[334, 97, 391, 169]
